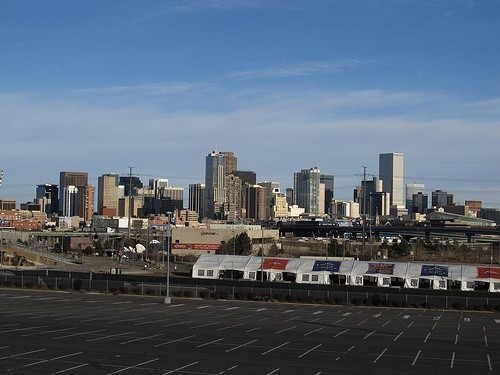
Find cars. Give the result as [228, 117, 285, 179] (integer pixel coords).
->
[278, 229, 465, 248]
[92, 249, 176, 272]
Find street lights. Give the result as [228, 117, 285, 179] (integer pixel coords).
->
[165, 211, 173, 304]
[230, 231, 235, 300]
